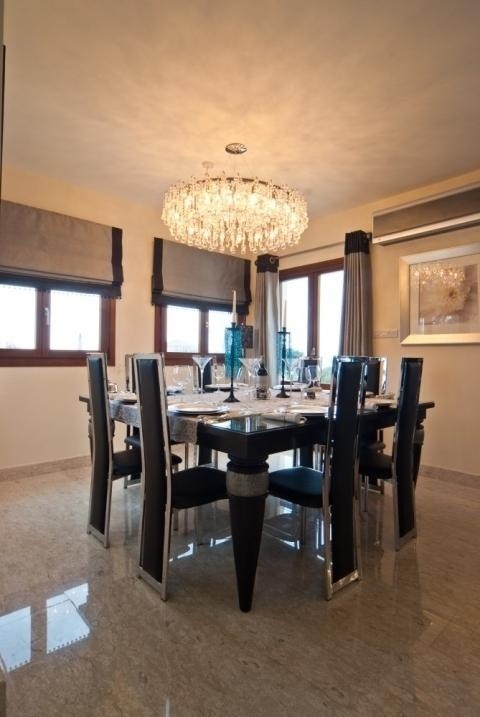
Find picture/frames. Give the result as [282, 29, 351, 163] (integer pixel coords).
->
[398, 243, 480, 346]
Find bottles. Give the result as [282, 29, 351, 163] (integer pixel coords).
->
[256, 363, 269, 400]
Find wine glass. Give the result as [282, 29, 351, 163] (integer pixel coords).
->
[192, 356, 213, 404]
[213, 363, 226, 394]
[239, 357, 262, 400]
[172, 364, 190, 403]
[281, 358, 321, 405]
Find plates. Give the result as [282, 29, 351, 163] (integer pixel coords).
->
[167, 404, 230, 415]
[288, 405, 329, 417]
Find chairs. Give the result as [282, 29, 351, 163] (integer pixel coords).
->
[361, 356, 422, 551]
[269, 356, 368, 600]
[133, 354, 229, 603]
[78, 353, 182, 549]
[314, 354, 386, 511]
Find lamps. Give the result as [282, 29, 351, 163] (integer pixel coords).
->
[159, 169, 309, 255]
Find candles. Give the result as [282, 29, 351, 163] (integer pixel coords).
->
[283, 298, 287, 328]
[231, 289, 238, 323]
